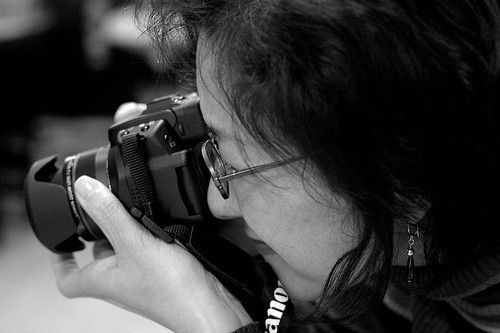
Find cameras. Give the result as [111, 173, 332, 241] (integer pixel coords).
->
[24, 92, 212, 255]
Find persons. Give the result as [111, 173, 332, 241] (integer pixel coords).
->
[51, 0, 500, 333]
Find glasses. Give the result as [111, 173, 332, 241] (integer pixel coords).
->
[201, 136, 310, 200]
